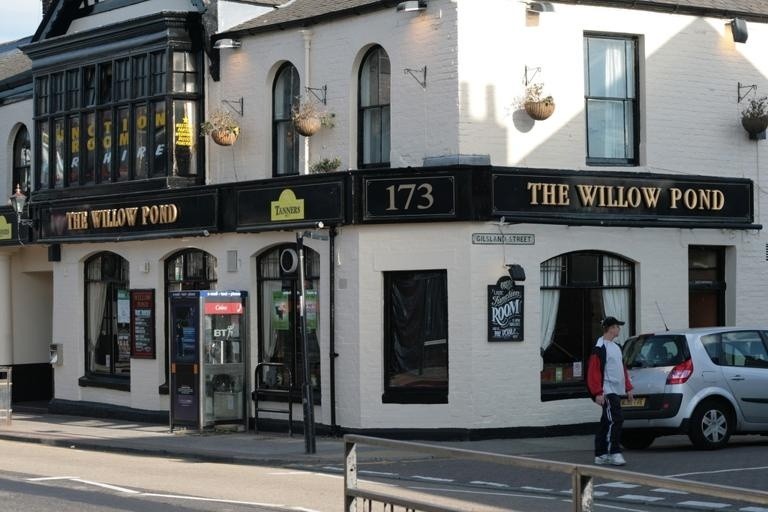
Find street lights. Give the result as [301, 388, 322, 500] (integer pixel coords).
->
[296, 229, 330, 453]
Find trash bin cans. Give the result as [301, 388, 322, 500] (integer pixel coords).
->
[0, 366, 13, 419]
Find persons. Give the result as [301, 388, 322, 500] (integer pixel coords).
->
[585, 316, 633, 466]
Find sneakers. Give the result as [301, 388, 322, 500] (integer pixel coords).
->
[594, 453, 611, 465]
[610, 453, 627, 465]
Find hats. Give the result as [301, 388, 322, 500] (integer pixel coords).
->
[602, 316, 625, 326]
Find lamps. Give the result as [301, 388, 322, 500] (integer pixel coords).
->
[397, 1, 427, 13]
[524, 1, 556, 15]
[214, 39, 241, 50]
[10, 183, 39, 231]
[726, 17, 748, 45]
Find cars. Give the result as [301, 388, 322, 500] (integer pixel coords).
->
[614, 298, 768, 453]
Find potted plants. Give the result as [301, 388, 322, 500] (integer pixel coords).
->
[290, 94, 322, 135]
[740, 94, 767, 135]
[520, 81, 555, 119]
[209, 107, 239, 145]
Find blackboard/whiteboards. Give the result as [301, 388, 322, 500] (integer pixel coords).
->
[488, 285, 524, 342]
